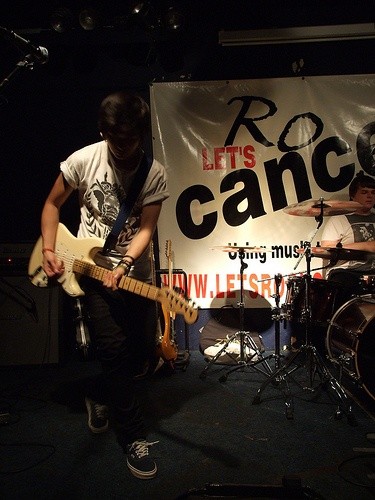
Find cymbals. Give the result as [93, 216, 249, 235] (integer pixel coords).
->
[295, 246, 375, 263]
[282, 198, 364, 217]
[208, 246, 276, 254]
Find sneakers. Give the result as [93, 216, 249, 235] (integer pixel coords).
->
[121, 444, 157, 479]
[85, 397, 109, 434]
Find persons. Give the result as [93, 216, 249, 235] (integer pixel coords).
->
[28, 94, 199, 480]
[321, 175, 375, 280]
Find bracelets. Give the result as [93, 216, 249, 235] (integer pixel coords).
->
[116, 255, 135, 276]
[42, 248, 55, 254]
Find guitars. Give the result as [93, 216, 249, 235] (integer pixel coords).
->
[160, 239, 181, 361]
[27, 221, 200, 325]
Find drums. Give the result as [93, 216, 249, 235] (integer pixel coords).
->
[325, 294, 375, 409]
[327, 269, 375, 297]
[284, 275, 337, 326]
